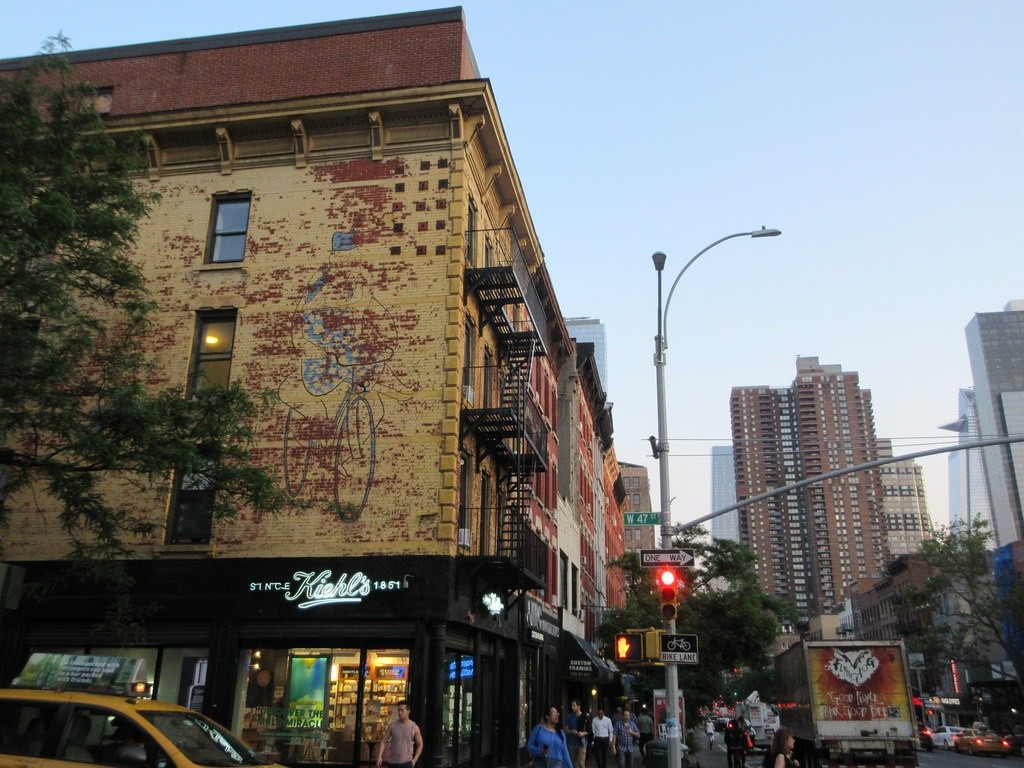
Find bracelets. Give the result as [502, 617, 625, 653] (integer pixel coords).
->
[612, 746, 616, 749]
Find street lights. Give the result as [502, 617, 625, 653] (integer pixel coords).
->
[652, 225, 782, 768]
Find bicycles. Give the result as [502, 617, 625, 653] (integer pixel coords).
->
[708, 733, 712, 751]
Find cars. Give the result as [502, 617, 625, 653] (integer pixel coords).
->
[707, 715, 731, 731]
[954, 728, 1009, 758]
[931, 726, 964, 750]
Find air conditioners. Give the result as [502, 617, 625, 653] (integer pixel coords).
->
[459, 528, 472, 548]
[581, 556, 586, 564]
[463, 385, 474, 407]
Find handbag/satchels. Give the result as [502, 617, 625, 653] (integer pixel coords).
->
[745, 732, 753, 747]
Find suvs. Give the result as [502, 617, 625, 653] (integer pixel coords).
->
[917, 721, 933, 753]
[0, 681, 292, 768]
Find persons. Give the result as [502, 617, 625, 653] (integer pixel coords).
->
[1004, 732, 1013, 745]
[953, 720, 959, 727]
[376, 700, 423, 768]
[564, 700, 592, 768]
[592, 708, 614, 768]
[762, 727, 799, 768]
[972, 718, 984, 729]
[724, 716, 756, 768]
[917, 721, 933, 748]
[704, 718, 715, 745]
[584, 704, 654, 760]
[612, 708, 640, 768]
[527, 706, 573, 768]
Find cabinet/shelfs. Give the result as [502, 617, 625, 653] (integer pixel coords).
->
[333, 679, 407, 733]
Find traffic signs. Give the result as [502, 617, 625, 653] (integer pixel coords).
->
[640, 549, 695, 567]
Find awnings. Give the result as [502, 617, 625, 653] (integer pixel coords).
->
[563, 631, 612, 672]
[454, 554, 543, 617]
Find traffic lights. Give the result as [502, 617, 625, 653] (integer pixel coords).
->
[615, 633, 643, 662]
[658, 567, 678, 618]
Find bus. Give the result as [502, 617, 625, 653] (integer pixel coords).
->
[735, 691, 781, 752]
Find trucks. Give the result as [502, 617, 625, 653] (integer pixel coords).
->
[773, 638, 920, 768]
